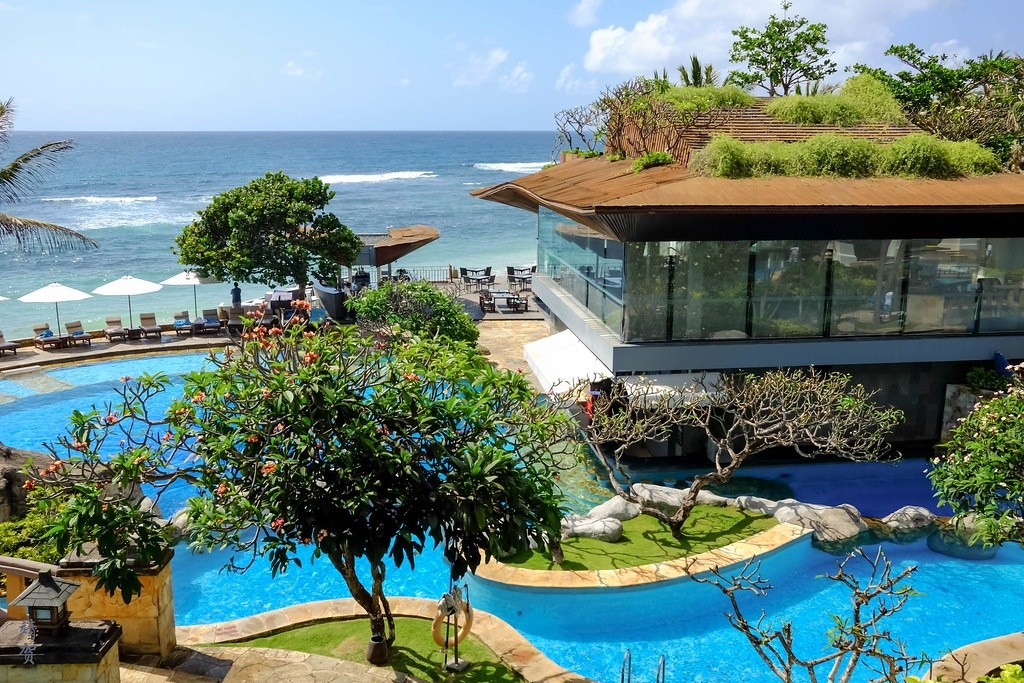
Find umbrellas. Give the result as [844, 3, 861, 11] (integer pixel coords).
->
[17, 281, 94, 336]
[91, 275, 163, 328]
[159, 268, 222, 318]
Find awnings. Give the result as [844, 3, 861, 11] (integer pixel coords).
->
[621, 372, 729, 410]
[523, 329, 614, 401]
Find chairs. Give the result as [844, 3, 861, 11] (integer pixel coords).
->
[459, 266, 537, 313]
[103, 316, 126, 343]
[202, 309, 221, 335]
[65, 320, 93, 347]
[31, 323, 63, 351]
[0, 330, 19, 357]
[228, 308, 245, 336]
[138, 313, 163, 340]
[174, 311, 194, 338]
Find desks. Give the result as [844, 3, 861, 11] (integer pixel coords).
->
[492, 294, 515, 312]
[191, 322, 205, 334]
[469, 276, 489, 292]
[128, 326, 142, 341]
[54, 334, 71, 348]
[513, 274, 532, 292]
[514, 266, 530, 274]
[466, 269, 485, 283]
[489, 289, 510, 308]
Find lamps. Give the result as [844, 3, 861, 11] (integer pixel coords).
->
[8, 569, 82, 637]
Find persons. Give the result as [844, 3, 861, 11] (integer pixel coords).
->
[230, 282, 242, 307]
[884, 291, 893, 319]
